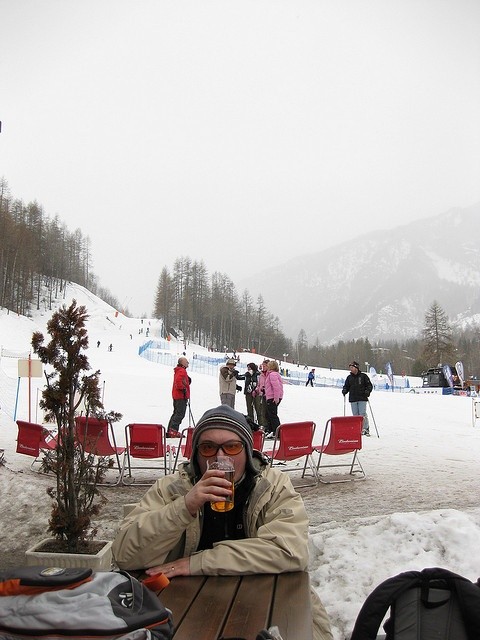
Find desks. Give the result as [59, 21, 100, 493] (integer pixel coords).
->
[126, 571, 313, 640]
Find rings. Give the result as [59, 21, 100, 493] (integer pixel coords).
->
[172, 567, 175, 571]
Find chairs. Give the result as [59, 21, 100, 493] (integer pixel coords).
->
[310, 416, 366, 484]
[251, 430, 265, 452]
[16, 420, 64, 479]
[122, 423, 172, 486]
[262, 421, 319, 489]
[74, 417, 125, 487]
[170, 428, 194, 474]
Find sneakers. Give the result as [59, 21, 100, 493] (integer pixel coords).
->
[362, 428, 369, 435]
[168, 428, 185, 438]
[265, 432, 275, 438]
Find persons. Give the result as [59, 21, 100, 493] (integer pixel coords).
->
[244, 363, 263, 428]
[265, 360, 284, 439]
[219, 357, 242, 409]
[342, 361, 373, 435]
[109, 343, 113, 352]
[168, 357, 192, 438]
[97, 340, 100, 347]
[111, 404, 334, 640]
[306, 369, 315, 387]
[252, 359, 271, 435]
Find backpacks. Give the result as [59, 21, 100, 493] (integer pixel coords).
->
[0, 567, 174, 639]
[350, 568, 480, 640]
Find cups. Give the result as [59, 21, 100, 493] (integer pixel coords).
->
[206, 456, 235, 512]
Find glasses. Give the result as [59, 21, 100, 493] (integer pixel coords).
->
[196, 440, 246, 457]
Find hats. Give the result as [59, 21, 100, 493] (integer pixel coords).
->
[190, 405, 260, 477]
[227, 359, 236, 366]
[349, 361, 359, 369]
[247, 363, 257, 371]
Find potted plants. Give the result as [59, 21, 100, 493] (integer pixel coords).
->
[25, 298, 123, 572]
